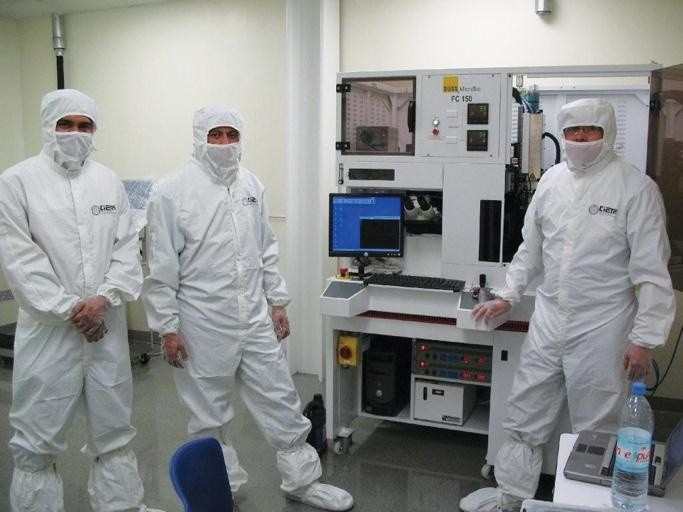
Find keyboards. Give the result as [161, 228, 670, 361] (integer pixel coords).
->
[365, 273, 466, 291]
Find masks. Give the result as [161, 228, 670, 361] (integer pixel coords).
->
[56, 132, 91, 157]
[564, 139, 602, 165]
[207, 142, 240, 168]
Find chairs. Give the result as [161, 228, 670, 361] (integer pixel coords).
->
[166, 436, 238, 511]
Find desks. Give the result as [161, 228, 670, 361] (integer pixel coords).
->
[552, 428, 680, 512]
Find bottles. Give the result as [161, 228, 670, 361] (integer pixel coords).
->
[609, 382, 654, 509]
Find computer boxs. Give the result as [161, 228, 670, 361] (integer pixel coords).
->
[362, 335, 411, 417]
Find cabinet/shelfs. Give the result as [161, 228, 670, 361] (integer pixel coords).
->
[319, 59, 660, 475]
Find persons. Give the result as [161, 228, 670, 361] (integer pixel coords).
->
[144, 103, 354, 512]
[459, 98, 677, 512]
[0, 89, 166, 511]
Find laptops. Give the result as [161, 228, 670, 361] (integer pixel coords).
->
[563, 415, 683, 496]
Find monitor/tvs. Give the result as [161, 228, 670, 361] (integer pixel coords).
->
[329, 193, 405, 275]
